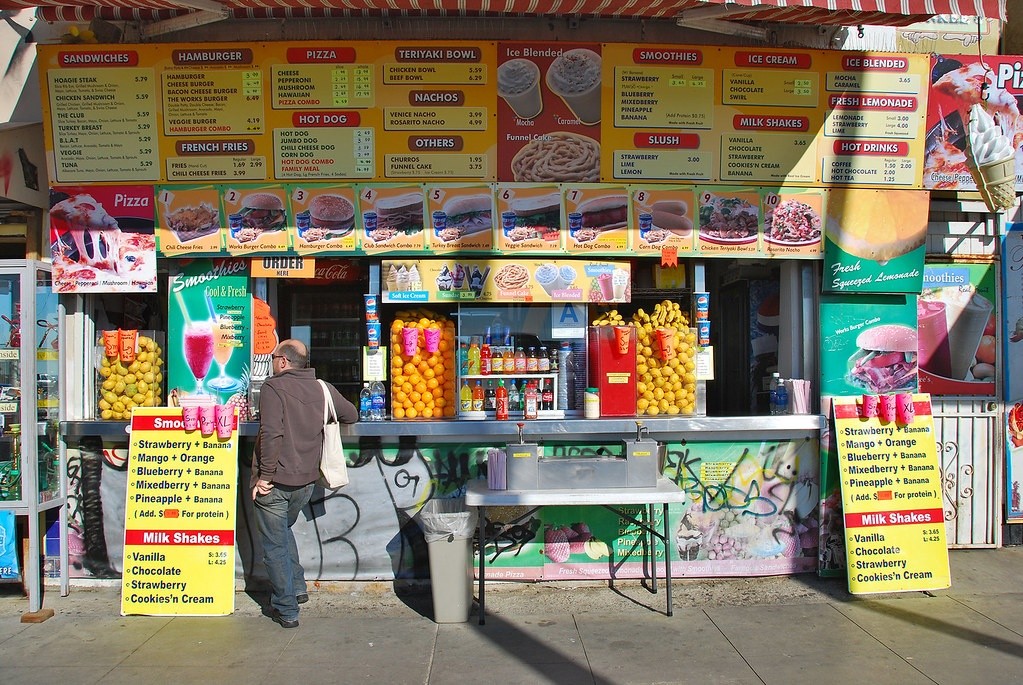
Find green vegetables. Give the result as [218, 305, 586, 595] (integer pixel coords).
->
[699, 198, 745, 225]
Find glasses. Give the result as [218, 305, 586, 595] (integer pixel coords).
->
[272, 354, 292, 362]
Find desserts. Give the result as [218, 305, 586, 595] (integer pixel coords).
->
[675, 514, 702, 562]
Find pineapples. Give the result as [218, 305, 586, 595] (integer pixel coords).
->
[225, 364, 251, 422]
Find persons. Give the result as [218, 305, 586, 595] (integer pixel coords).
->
[250, 339, 358, 629]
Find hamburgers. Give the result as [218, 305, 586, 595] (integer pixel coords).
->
[485, 506, 541, 556]
[309, 195, 355, 240]
[241, 193, 287, 233]
[846, 324, 918, 391]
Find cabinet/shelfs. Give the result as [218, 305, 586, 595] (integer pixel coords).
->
[0, 258, 69, 613]
[452, 303, 589, 421]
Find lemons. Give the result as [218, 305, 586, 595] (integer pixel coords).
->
[100, 336, 164, 419]
[636, 319, 696, 415]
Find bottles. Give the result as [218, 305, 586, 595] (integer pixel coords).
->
[583, 387, 600, 419]
[296, 295, 360, 316]
[361, 313, 559, 420]
[312, 322, 360, 383]
[770, 372, 780, 415]
[776, 378, 788, 414]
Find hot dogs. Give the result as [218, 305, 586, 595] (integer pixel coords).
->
[509, 192, 560, 234]
[375, 192, 423, 235]
[442, 194, 492, 235]
[576, 196, 628, 231]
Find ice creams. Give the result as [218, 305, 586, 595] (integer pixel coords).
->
[964, 104, 1015, 211]
[435, 262, 491, 297]
[386, 264, 420, 291]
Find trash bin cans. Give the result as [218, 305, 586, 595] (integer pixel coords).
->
[418, 497, 477, 624]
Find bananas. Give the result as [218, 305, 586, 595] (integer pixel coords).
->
[592, 310, 625, 325]
[584, 536, 614, 559]
[628, 300, 691, 334]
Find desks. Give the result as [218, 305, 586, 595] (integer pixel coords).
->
[466, 478, 685, 616]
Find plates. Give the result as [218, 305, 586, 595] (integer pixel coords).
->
[699, 231, 758, 245]
[764, 234, 822, 245]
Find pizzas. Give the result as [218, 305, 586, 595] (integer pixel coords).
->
[924, 61, 1023, 190]
[49, 194, 157, 292]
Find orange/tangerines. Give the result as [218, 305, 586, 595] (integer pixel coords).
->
[391, 310, 456, 418]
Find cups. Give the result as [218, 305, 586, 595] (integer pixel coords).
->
[940, 291, 994, 381]
[862, 394, 878, 418]
[365, 212, 517, 238]
[895, 393, 914, 424]
[102, 329, 137, 361]
[614, 292, 711, 360]
[498, 49, 601, 126]
[879, 394, 897, 421]
[569, 214, 653, 238]
[183, 405, 235, 438]
[560, 340, 586, 410]
[364, 294, 381, 348]
[401, 327, 418, 357]
[423, 327, 440, 352]
[436, 263, 625, 300]
[918, 302, 952, 379]
[229, 214, 311, 237]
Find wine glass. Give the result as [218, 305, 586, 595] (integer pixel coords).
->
[183, 320, 243, 397]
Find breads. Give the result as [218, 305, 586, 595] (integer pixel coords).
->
[649, 201, 694, 236]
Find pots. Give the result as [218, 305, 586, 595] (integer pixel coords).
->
[925, 94, 1022, 171]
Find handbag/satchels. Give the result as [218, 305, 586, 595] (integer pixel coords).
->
[317, 379, 348, 488]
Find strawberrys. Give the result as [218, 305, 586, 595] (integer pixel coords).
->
[544, 522, 593, 562]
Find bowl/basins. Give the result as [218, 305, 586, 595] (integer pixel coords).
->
[52, 216, 154, 264]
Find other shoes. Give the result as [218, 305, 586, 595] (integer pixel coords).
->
[297, 594, 309, 604]
[273, 611, 299, 628]
